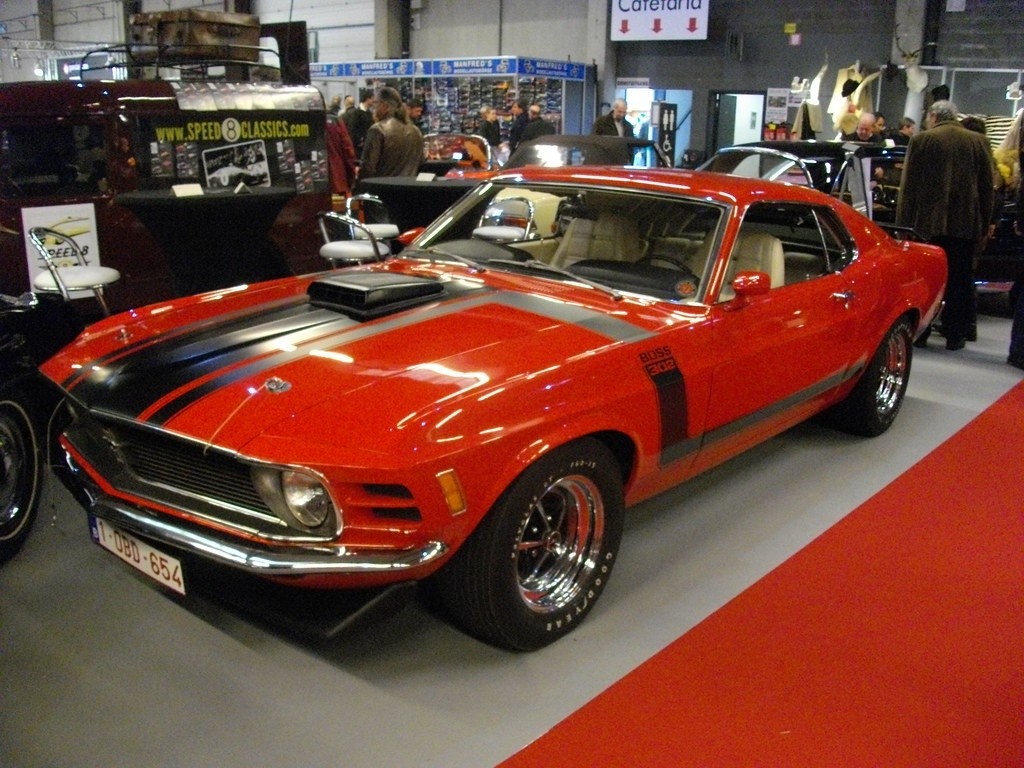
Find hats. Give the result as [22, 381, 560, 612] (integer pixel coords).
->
[906, 64, 928, 92]
[932, 84, 950, 102]
[841, 79, 860, 97]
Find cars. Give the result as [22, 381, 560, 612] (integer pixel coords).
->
[691, 140, 1024, 305]
[0, 294, 59, 556]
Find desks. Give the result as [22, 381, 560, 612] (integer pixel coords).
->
[359, 177, 496, 256]
[115, 186, 296, 297]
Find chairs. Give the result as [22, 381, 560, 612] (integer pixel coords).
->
[29, 226, 121, 320]
[551, 215, 650, 270]
[317, 210, 390, 270]
[344, 193, 400, 242]
[689, 226, 785, 303]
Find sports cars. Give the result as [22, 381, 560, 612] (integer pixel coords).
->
[35, 165, 949, 651]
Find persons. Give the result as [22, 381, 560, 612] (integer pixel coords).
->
[477, 98, 649, 166]
[325, 87, 422, 249]
[841, 99, 1024, 367]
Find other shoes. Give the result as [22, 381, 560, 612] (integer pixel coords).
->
[914, 338, 927, 348]
[1006, 353, 1024, 370]
[960, 324, 978, 341]
[946, 334, 966, 351]
